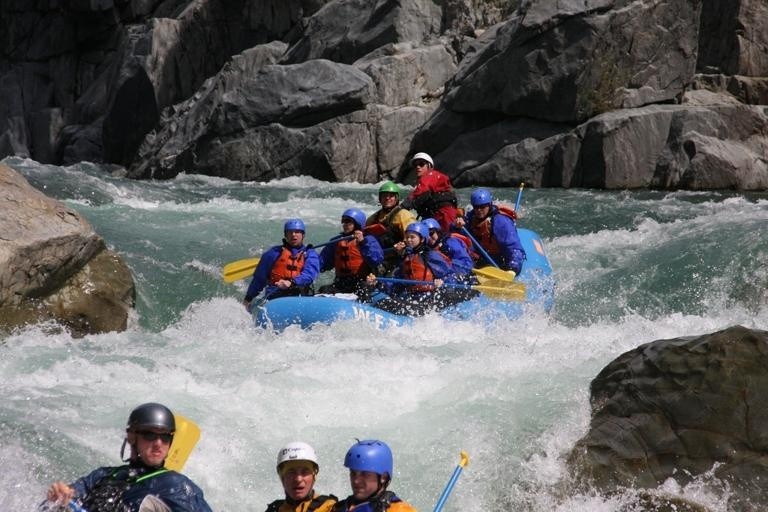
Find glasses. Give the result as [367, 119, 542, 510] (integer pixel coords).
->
[413, 162, 430, 168]
[136, 429, 174, 443]
[341, 218, 353, 223]
[429, 229, 435, 236]
[474, 204, 489, 209]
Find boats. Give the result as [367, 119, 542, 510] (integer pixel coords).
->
[251, 226, 554, 343]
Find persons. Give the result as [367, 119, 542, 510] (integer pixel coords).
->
[36, 402, 214, 512]
[243, 217, 322, 304]
[262, 441, 339, 512]
[331, 436, 423, 512]
[319, 153, 527, 317]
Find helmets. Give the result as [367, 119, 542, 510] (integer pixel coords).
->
[405, 222, 428, 245]
[471, 188, 493, 206]
[378, 181, 400, 202]
[341, 208, 366, 229]
[409, 152, 434, 168]
[421, 217, 441, 233]
[126, 402, 176, 432]
[284, 219, 304, 232]
[344, 439, 393, 482]
[276, 441, 319, 466]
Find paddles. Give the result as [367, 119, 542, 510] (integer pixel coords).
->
[165, 416, 199, 472]
[224, 235, 355, 283]
[367, 276, 526, 301]
[403, 246, 514, 284]
